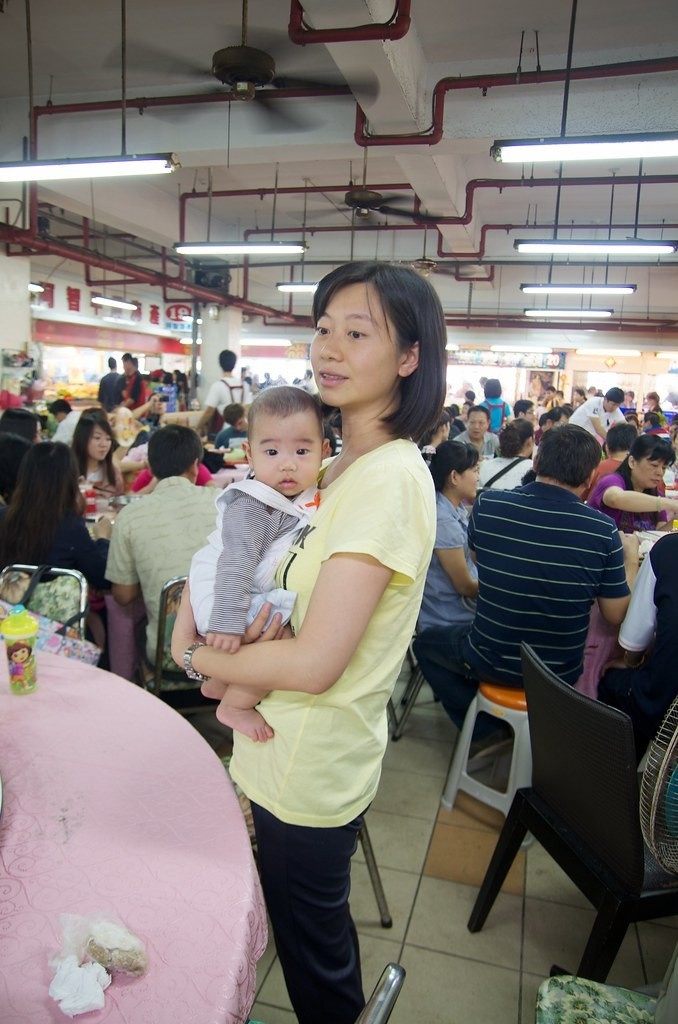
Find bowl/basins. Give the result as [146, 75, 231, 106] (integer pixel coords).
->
[109, 495, 143, 513]
[632, 529, 669, 544]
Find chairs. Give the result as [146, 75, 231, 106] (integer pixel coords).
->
[466, 638, 678, 986]
[410, 618, 526, 748]
[135, 574, 249, 714]
[535, 940, 678, 1024]
[217, 753, 394, 930]
[0, 559, 88, 639]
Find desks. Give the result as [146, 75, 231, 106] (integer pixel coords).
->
[0, 637, 269, 1024]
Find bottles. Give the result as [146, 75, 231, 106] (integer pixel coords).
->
[1, 605, 38, 695]
[669, 520, 678, 533]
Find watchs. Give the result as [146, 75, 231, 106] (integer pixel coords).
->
[184, 642, 211, 682]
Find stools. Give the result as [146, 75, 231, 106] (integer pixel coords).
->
[438, 683, 536, 849]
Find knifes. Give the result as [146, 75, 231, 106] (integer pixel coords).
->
[620, 521, 662, 538]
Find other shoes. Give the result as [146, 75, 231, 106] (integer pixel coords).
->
[466, 732, 513, 774]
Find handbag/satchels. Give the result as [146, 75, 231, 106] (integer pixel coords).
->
[0, 565, 103, 667]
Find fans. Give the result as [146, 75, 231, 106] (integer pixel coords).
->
[287, 120, 445, 222]
[99, 0, 381, 135]
[629, 689, 678, 998]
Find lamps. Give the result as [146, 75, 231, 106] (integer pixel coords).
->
[28, 282, 44, 293]
[489, 0, 678, 165]
[523, 266, 614, 318]
[172, 160, 309, 255]
[276, 223, 382, 294]
[512, 156, 678, 255]
[520, 262, 637, 295]
[0, 0, 181, 184]
[90, 224, 138, 312]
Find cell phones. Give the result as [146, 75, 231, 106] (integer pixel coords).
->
[159, 395, 170, 401]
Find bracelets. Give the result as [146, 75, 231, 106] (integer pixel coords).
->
[624, 651, 644, 667]
[657, 496, 661, 513]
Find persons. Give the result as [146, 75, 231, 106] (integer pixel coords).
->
[189, 384, 329, 741]
[168, 261, 448, 1024]
[0, 350, 314, 671]
[417, 439, 478, 633]
[598, 531, 678, 760]
[587, 433, 678, 534]
[418, 377, 678, 514]
[412, 423, 639, 759]
[104, 425, 225, 705]
